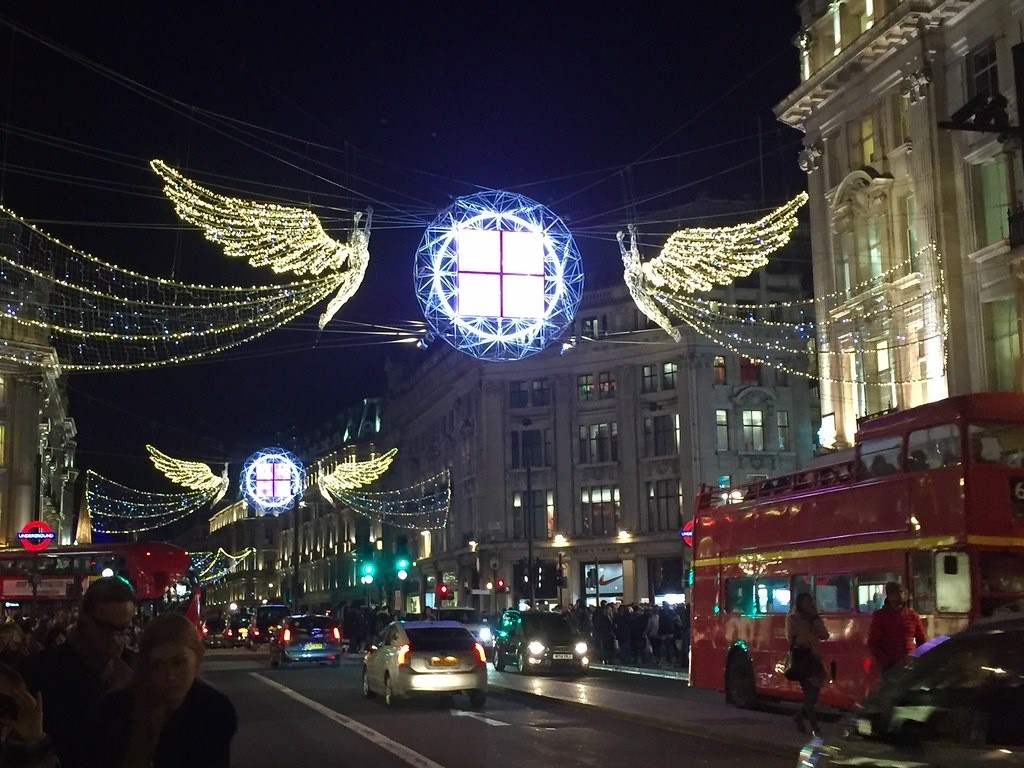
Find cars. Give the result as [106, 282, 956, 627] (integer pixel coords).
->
[492, 610, 589, 676]
[794, 614, 1024, 768]
[362, 620, 487, 707]
[200, 612, 254, 649]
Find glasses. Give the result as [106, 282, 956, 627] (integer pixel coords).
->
[86, 614, 135, 638]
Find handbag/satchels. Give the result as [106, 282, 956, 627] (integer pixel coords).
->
[783, 636, 820, 683]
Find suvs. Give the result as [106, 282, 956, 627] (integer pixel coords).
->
[249, 605, 291, 647]
[269, 615, 341, 670]
[435, 607, 495, 660]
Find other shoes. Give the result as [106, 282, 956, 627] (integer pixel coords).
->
[792, 715, 806, 733]
[808, 727, 822, 738]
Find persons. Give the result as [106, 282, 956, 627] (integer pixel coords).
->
[55, 613, 239, 768]
[368, 605, 398, 635]
[0, 662, 61, 768]
[744, 436, 983, 501]
[868, 582, 927, 671]
[420, 607, 437, 622]
[554, 598, 690, 668]
[0, 575, 136, 731]
[786, 593, 830, 734]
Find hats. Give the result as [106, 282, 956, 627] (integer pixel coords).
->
[885, 581, 901, 595]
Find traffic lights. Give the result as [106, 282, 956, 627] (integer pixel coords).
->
[497, 579, 505, 592]
[440, 585, 447, 600]
[397, 548, 409, 572]
[362, 556, 374, 576]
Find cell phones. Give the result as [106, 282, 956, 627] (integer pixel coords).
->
[0, 694, 19, 721]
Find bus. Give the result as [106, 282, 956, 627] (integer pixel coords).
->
[0, 541, 203, 642]
[688, 392, 1023, 713]
